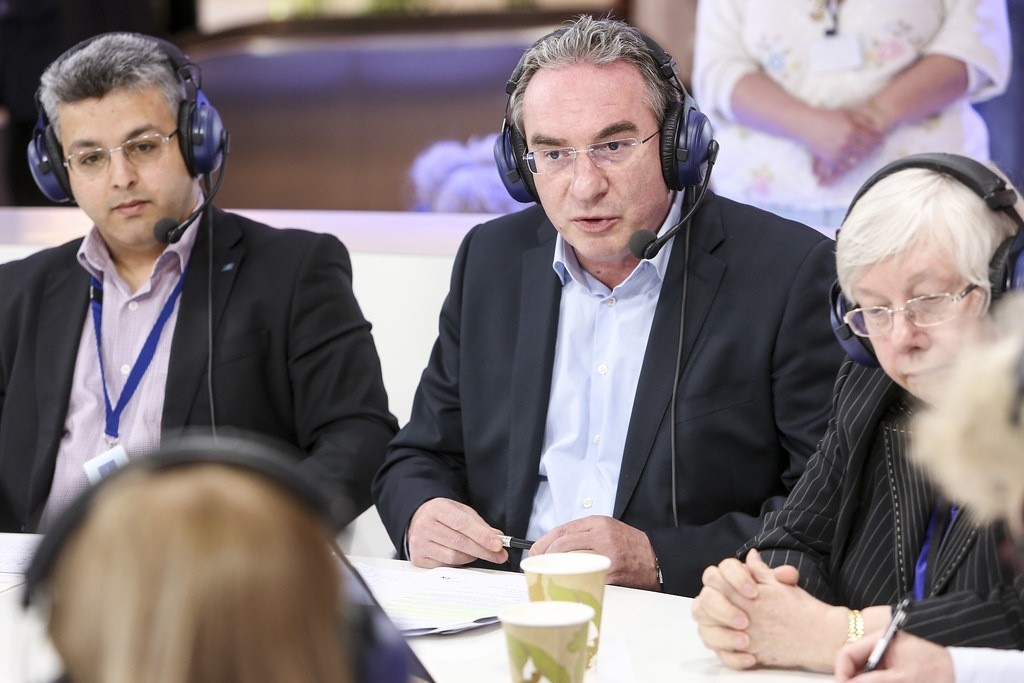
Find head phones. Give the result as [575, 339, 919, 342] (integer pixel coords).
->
[494, 27, 719, 210]
[27, 32, 229, 204]
[828, 152, 1023, 369]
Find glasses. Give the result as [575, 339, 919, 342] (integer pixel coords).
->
[843, 283, 978, 337]
[522, 128, 663, 175]
[62, 127, 179, 178]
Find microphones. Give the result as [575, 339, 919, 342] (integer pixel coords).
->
[152, 154, 228, 245]
[627, 160, 712, 261]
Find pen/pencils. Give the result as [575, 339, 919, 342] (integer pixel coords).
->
[863, 591, 915, 673]
[496, 533, 535, 551]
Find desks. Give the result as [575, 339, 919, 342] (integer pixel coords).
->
[0, 531, 842, 683]
[1, 207, 512, 450]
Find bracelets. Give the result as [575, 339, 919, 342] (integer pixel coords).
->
[656, 557, 663, 586]
[846, 609, 864, 642]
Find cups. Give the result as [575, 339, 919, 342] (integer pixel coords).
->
[520, 553, 610, 666]
[496, 600, 594, 683]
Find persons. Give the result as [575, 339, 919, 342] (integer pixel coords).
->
[691, 0, 1014, 239]
[0, 32, 399, 540]
[834, 340, 1024, 683]
[693, 150, 1024, 672]
[371, 15, 843, 597]
[18, 433, 436, 683]
